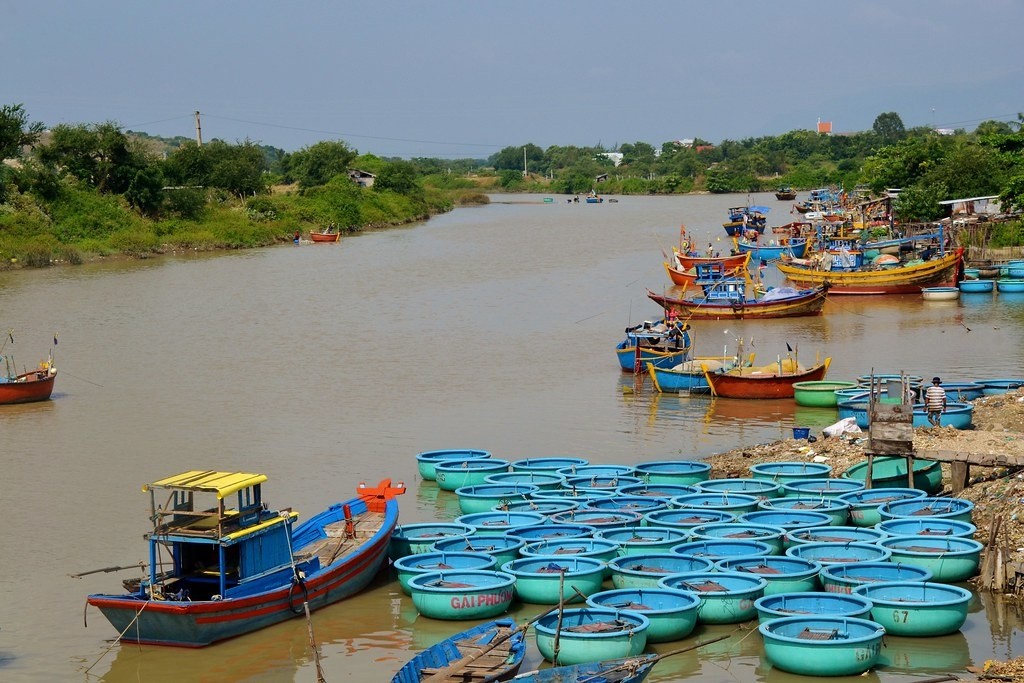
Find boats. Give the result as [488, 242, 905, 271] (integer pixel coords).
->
[646, 336, 756, 395]
[614, 307, 694, 374]
[643, 260, 834, 322]
[498, 653, 661, 683]
[310, 220, 341, 242]
[390, 615, 527, 683]
[585, 193, 603, 203]
[543, 197, 553, 203]
[662, 189, 1024, 303]
[68, 468, 408, 649]
[389, 370, 1024, 678]
[0, 328, 62, 407]
[699, 336, 832, 400]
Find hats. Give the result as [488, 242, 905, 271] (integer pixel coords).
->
[932, 377, 942, 384]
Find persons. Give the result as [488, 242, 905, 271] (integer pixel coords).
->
[328, 224, 334, 233]
[294, 229, 300, 243]
[897, 252, 925, 269]
[706, 243, 719, 258]
[923, 377, 946, 426]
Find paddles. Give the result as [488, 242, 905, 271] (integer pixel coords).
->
[606, 633, 732, 683]
[420, 584, 589, 683]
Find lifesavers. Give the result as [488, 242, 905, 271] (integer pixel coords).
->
[287, 580, 308, 615]
